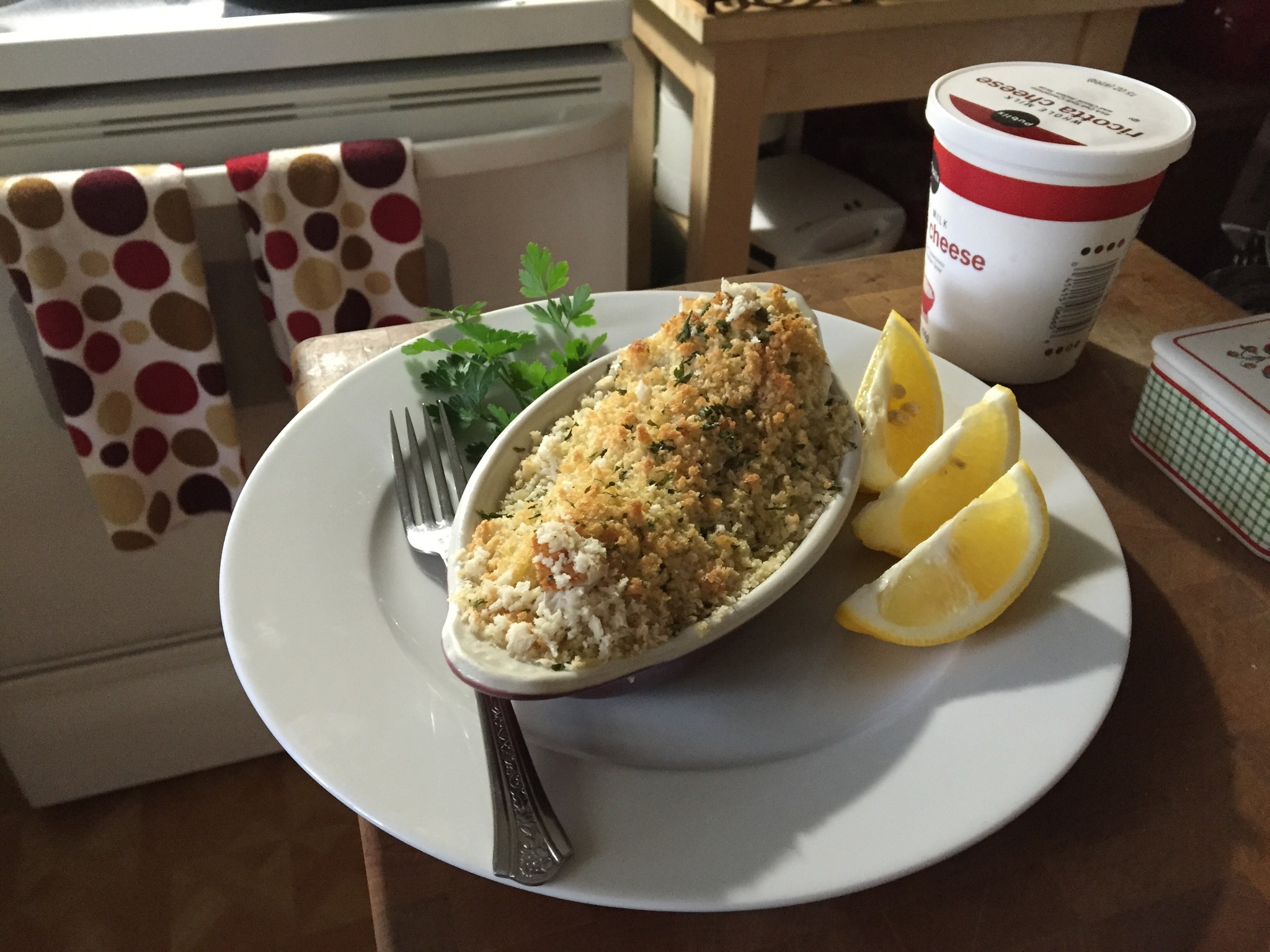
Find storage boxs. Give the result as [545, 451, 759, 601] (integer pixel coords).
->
[1128, 312, 1269, 562]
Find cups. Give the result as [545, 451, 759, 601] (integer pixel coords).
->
[918, 62, 1195, 383]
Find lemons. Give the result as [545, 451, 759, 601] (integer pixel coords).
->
[851, 309, 945, 495]
[831, 458, 1050, 648]
[851, 382, 1022, 559]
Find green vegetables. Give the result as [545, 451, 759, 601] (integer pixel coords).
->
[395, 241, 611, 469]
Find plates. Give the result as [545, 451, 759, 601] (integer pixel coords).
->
[441, 282, 862, 700]
[217, 291, 1133, 914]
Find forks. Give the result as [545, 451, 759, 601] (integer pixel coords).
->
[387, 401, 573, 887]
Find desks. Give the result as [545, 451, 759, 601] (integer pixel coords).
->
[625, 0, 1180, 297]
[292, 242, 1269, 952]
